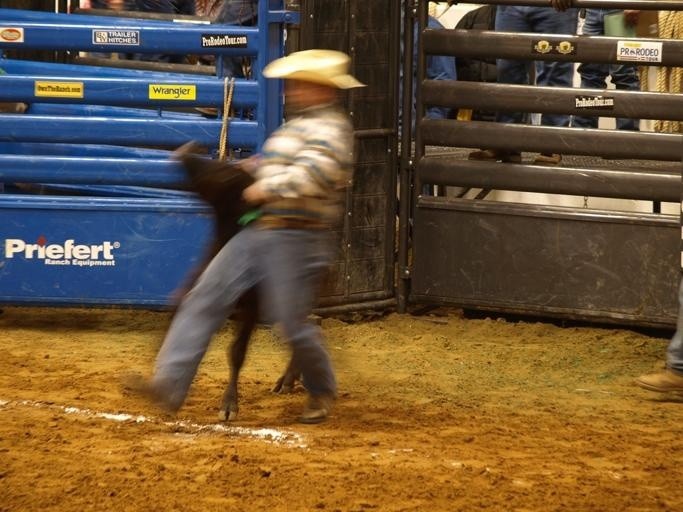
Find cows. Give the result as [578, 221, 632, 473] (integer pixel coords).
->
[170, 138, 340, 420]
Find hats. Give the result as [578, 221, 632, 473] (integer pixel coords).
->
[261, 48, 370, 90]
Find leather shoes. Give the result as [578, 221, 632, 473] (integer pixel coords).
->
[468, 148, 514, 161]
[534, 149, 562, 164]
[635, 370, 682, 392]
[301, 393, 331, 423]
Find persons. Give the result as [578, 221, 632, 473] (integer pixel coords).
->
[397, 2, 641, 168]
[120, 46, 367, 425]
[634, 279, 683, 394]
[0, 0, 256, 122]
